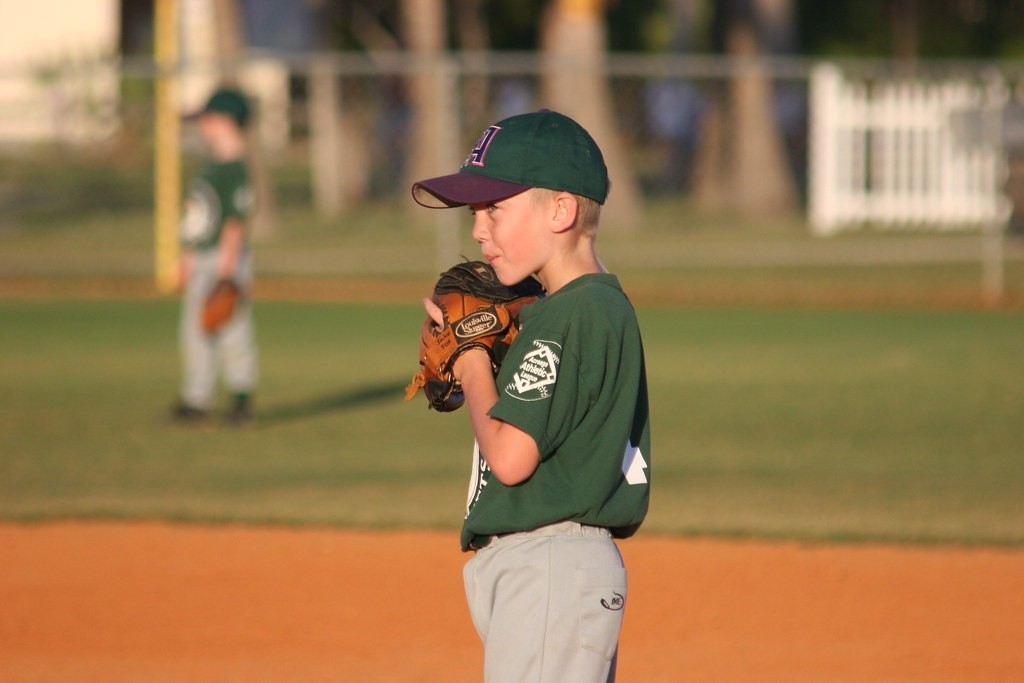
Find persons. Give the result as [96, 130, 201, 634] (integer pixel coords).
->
[172, 87, 257, 422]
[412, 111, 652, 683]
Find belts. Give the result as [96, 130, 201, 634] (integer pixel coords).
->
[466, 524, 599, 548]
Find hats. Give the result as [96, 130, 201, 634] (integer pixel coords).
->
[410, 108, 610, 207]
[179, 89, 249, 130]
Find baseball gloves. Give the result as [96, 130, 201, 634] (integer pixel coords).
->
[419, 259, 547, 415]
